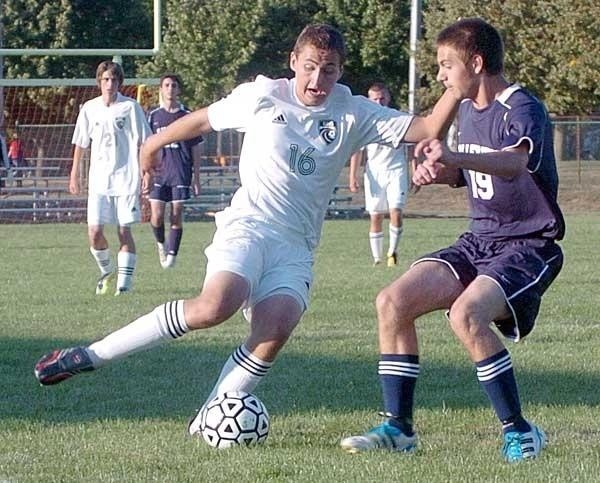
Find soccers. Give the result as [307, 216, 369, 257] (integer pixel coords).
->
[197, 391, 270, 449]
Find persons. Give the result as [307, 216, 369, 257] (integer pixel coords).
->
[349, 85, 408, 268]
[142, 75, 205, 268]
[338, 18, 566, 463]
[33, 25, 460, 435]
[68, 62, 153, 296]
[8, 133, 26, 188]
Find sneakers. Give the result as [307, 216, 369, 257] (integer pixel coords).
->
[375, 261, 384, 268]
[117, 288, 130, 297]
[504, 425, 543, 466]
[159, 244, 166, 266]
[388, 253, 400, 268]
[96, 270, 114, 296]
[163, 260, 175, 271]
[35, 346, 95, 386]
[190, 412, 203, 438]
[342, 424, 418, 455]
